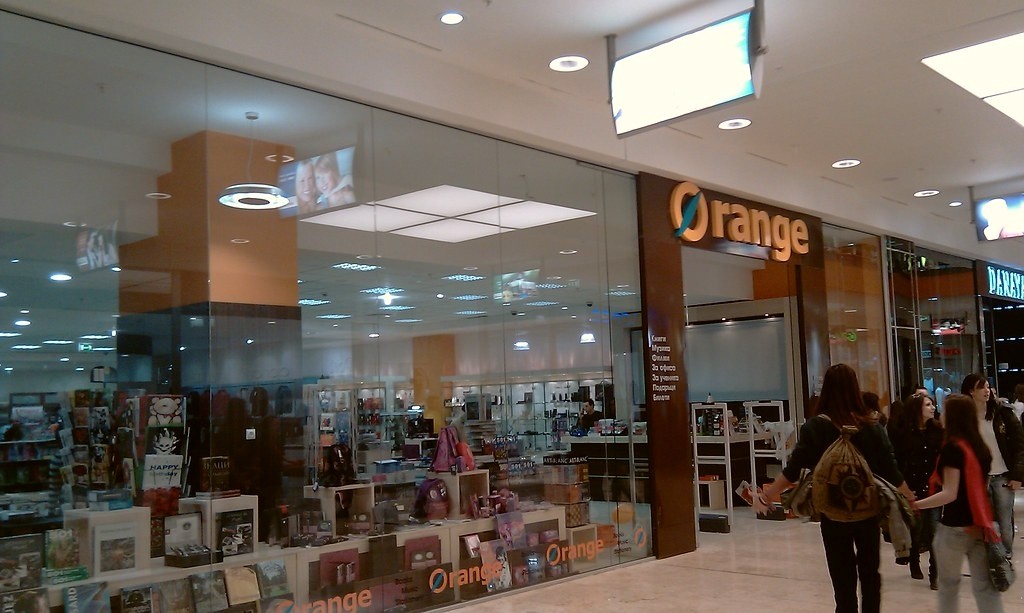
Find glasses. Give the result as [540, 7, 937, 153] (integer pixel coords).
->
[912, 392, 922, 398]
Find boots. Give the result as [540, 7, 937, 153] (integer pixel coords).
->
[927, 547, 940, 591]
[830, 569, 859, 613]
[909, 540, 925, 579]
[858, 569, 882, 613]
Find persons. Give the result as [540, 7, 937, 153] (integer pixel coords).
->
[89, 230, 120, 269]
[1012, 383, 1024, 421]
[784, 363, 918, 613]
[576, 399, 603, 433]
[892, 392, 945, 590]
[861, 391, 888, 437]
[990, 386, 998, 401]
[914, 386, 929, 396]
[959, 373, 1024, 572]
[907, 392, 1008, 613]
[295, 151, 356, 214]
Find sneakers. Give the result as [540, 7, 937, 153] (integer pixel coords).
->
[1005, 550, 1013, 560]
[895, 557, 909, 565]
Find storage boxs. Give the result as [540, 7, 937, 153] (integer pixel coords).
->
[45, 463, 598, 613]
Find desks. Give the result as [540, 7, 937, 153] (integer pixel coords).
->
[561, 431, 774, 508]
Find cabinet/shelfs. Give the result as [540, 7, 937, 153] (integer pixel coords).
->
[301, 371, 615, 471]
[0, 388, 123, 534]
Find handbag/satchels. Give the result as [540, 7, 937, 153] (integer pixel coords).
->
[984, 536, 1017, 593]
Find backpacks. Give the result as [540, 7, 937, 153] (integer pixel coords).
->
[413, 478, 450, 525]
[809, 413, 883, 523]
[428, 425, 460, 472]
[319, 443, 355, 488]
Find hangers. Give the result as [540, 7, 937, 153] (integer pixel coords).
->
[213, 381, 289, 403]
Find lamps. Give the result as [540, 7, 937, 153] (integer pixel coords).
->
[218, 109, 291, 211]
[513, 312, 530, 351]
[579, 301, 596, 344]
